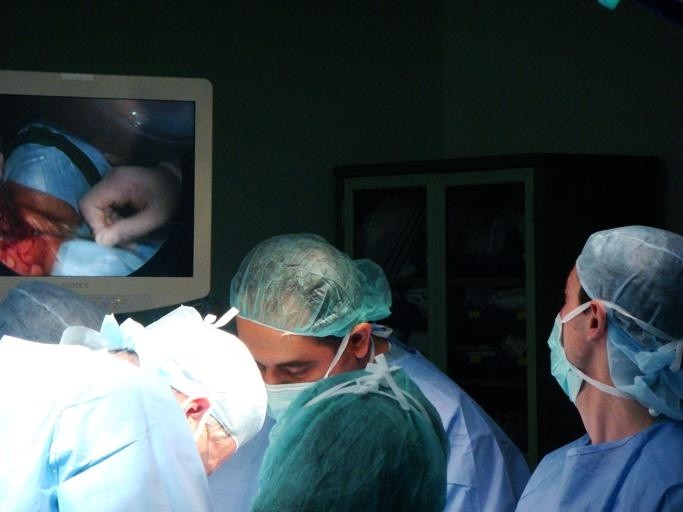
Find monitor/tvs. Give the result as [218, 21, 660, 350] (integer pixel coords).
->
[0, 70, 214, 315]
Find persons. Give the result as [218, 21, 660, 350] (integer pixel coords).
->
[227, 231, 531, 510]
[0, 180, 82, 277]
[78, 158, 193, 246]
[0, 302, 269, 510]
[511, 224, 683, 510]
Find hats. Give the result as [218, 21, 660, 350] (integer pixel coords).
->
[103, 304, 268, 450]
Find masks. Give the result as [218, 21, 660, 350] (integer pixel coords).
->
[268, 340, 377, 421]
[547, 304, 596, 402]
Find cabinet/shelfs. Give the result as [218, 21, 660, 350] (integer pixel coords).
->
[334, 151, 665, 466]
